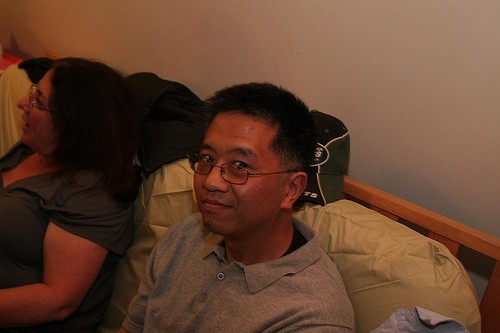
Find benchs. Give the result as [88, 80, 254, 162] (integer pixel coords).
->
[0, 46, 500, 333]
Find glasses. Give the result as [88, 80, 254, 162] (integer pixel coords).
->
[27, 84, 57, 114]
[183, 154, 303, 185]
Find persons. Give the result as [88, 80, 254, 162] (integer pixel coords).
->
[119, 82, 356, 333]
[0, 57, 134, 333]
